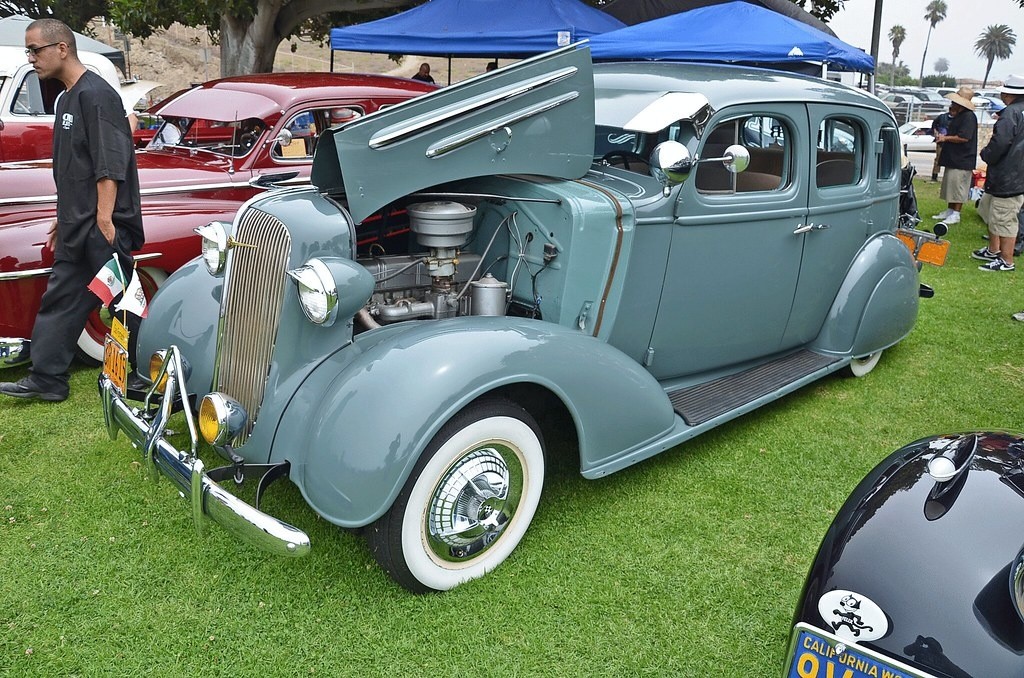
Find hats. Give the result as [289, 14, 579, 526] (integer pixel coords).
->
[996, 74, 1024, 94]
[943, 87, 976, 111]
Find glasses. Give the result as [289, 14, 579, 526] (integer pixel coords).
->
[24, 42, 69, 58]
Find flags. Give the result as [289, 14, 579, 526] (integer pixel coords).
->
[113, 268, 148, 319]
[87, 258, 129, 308]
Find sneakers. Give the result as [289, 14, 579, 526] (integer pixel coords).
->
[0, 375, 68, 401]
[972, 246, 1002, 262]
[127, 370, 148, 390]
[978, 257, 1015, 273]
[942, 211, 961, 224]
[932, 211, 949, 219]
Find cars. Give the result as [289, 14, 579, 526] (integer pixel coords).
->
[899, 122, 936, 151]
[0, 46, 163, 163]
[781, 429, 1024, 678]
[96, 41, 950, 595]
[0, 73, 443, 368]
[744, 115, 856, 152]
[858, 81, 1007, 119]
[973, 109, 997, 127]
[881, 93, 924, 116]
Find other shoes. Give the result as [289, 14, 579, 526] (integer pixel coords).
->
[1013, 250, 1021, 256]
[982, 234, 990, 241]
[1011, 310, 1024, 323]
[4, 341, 31, 364]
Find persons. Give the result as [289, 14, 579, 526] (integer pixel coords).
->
[930, 74, 1024, 271]
[0, 19, 141, 401]
[932, 86, 978, 224]
[486, 61, 498, 71]
[412, 63, 435, 85]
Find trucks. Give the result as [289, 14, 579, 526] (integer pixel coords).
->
[827, 71, 865, 87]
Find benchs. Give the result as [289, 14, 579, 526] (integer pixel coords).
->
[697, 166, 782, 192]
[746, 146, 856, 187]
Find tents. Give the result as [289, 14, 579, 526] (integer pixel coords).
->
[328, 0, 629, 72]
[576, 0, 876, 96]
